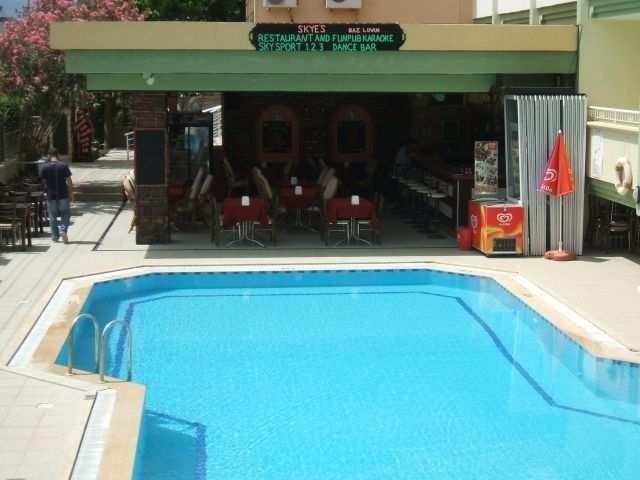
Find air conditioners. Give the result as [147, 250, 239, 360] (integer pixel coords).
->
[263, 0, 363, 9]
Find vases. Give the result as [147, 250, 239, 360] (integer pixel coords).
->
[20, 157, 52, 182]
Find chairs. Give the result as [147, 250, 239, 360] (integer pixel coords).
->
[0, 176, 45, 249]
[122, 167, 137, 232]
[177, 155, 386, 247]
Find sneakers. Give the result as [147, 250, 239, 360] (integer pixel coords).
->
[52, 231, 68, 243]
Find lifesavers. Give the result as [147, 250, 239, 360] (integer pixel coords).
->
[614, 157, 633, 195]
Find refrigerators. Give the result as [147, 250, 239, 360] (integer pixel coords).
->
[474, 136, 505, 196]
[468, 198, 524, 257]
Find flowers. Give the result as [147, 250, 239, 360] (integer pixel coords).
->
[1, 0, 145, 159]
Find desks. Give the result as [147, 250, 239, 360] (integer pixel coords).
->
[169, 175, 184, 234]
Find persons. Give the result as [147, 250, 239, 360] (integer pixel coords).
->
[41, 148, 73, 242]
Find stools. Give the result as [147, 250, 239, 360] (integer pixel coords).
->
[391, 176, 448, 237]
